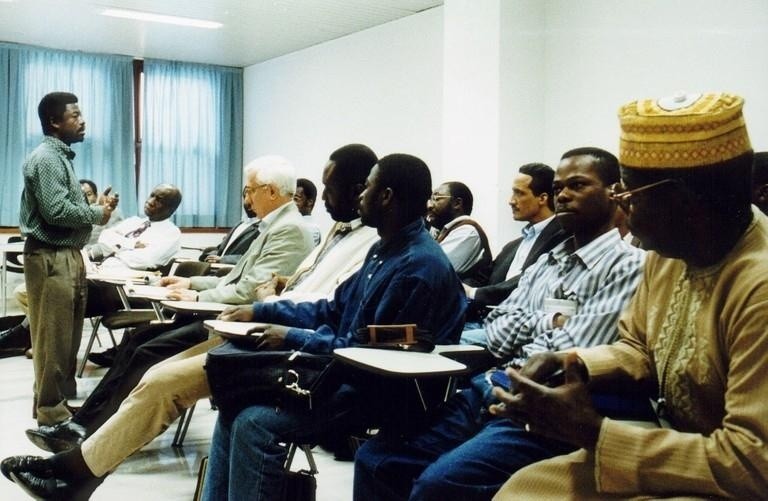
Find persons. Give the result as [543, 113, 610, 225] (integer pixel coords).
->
[0, 144, 379, 499]
[20, 91, 119, 432]
[77, 180, 123, 249]
[24, 154, 316, 455]
[200, 88, 767, 501]
[294, 178, 321, 247]
[87, 186, 262, 366]
[0, 182, 182, 348]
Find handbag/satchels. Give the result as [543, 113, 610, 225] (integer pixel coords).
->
[194, 455, 317, 501]
[206, 337, 336, 411]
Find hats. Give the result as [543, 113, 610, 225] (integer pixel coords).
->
[620, 92, 752, 168]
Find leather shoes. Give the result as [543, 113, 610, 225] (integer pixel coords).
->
[25, 424, 86, 453]
[1, 455, 77, 501]
[88, 349, 115, 366]
[0, 328, 28, 348]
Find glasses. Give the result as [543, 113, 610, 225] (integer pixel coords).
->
[430, 195, 453, 203]
[609, 180, 678, 216]
[244, 184, 266, 195]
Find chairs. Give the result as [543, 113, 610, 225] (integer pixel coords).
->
[0, 231, 488, 501]
